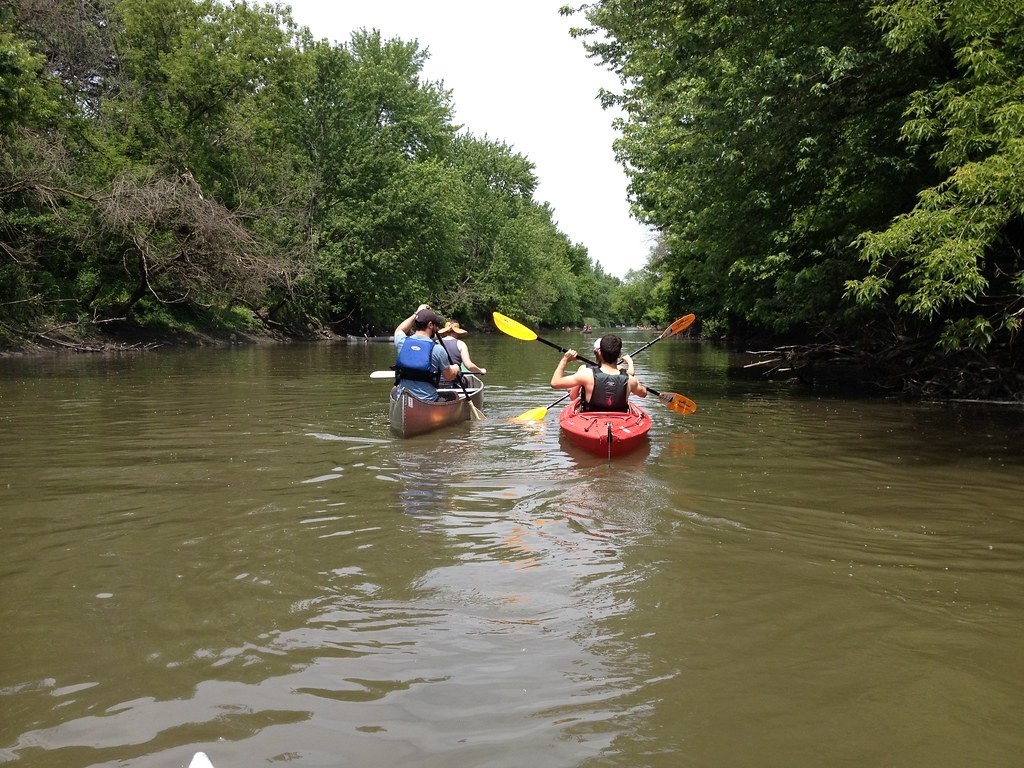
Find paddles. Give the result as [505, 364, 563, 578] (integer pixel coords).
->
[493, 310, 698, 417]
[436, 332, 487, 422]
[368, 369, 486, 379]
[509, 313, 696, 423]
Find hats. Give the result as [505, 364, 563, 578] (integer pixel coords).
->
[438, 322, 467, 334]
[593, 338, 601, 351]
[414, 306, 443, 322]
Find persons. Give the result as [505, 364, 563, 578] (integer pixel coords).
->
[550, 334, 647, 419]
[433, 319, 487, 389]
[568, 337, 636, 400]
[393, 303, 461, 402]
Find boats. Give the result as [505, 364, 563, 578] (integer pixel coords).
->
[388, 371, 484, 438]
[558, 394, 655, 459]
